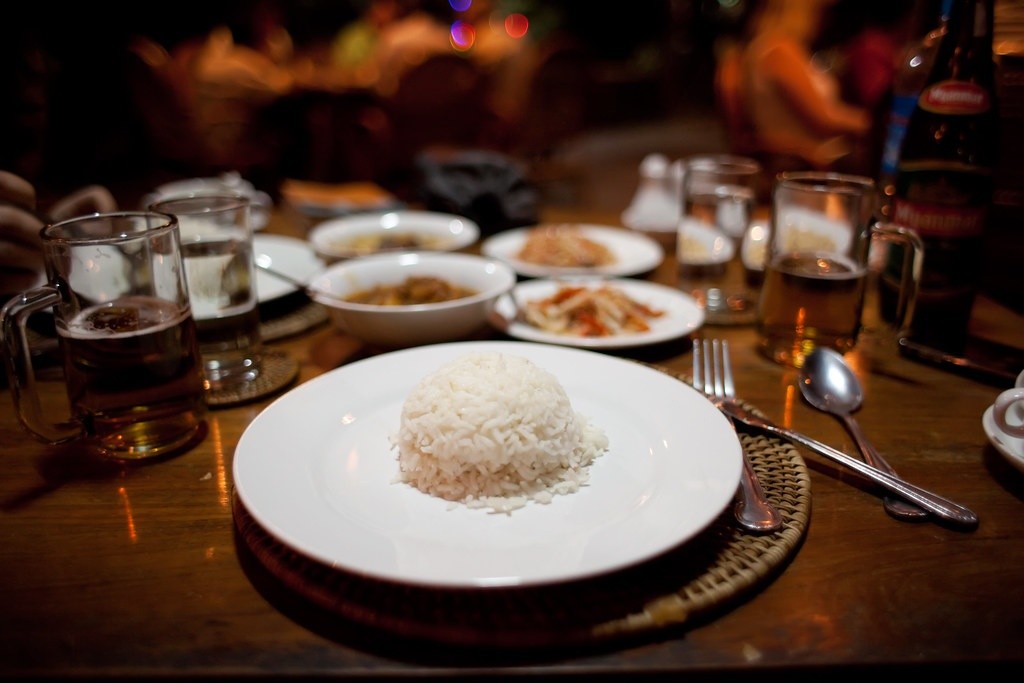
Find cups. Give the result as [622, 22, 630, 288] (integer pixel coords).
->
[671, 153, 762, 327]
[755, 168, 927, 369]
[0, 210, 210, 462]
[156, 177, 256, 244]
[993, 370, 1024, 439]
[146, 194, 263, 390]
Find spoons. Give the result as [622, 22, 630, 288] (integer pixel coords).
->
[797, 346, 925, 521]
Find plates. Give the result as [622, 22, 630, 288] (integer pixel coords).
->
[305, 208, 478, 259]
[981, 398, 1024, 468]
[488, 275, 702, 349]
[232, 338, 746, 591]
[41, 230, 324, 319]
[479, 221, 664, 278]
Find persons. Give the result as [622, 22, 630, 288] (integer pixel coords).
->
[0, 1, 217, 315]
[735, 1, 871, 178]
[152, 0, 490, 195]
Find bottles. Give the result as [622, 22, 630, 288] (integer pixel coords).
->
[868, 0, 1000, 344]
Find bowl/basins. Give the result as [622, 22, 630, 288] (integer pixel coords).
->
[307, 250, 515, 338]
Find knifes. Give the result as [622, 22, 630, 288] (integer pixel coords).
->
[713, 399, 978, 526]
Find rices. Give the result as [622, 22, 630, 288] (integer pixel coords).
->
[393, 350, 608, 511]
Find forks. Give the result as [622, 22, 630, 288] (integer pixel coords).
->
[687, 338, 783, 531]
